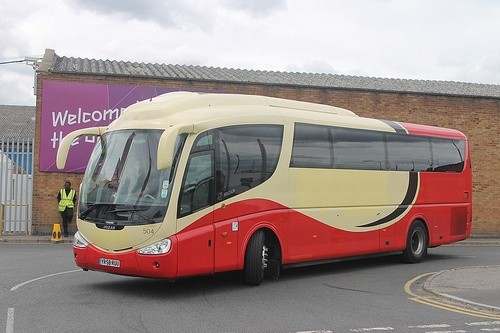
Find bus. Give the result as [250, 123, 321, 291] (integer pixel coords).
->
[55, 91, 473, 287]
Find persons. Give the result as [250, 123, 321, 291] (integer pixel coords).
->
[57, 181, 76, 237]
[111, 159, 160, 202]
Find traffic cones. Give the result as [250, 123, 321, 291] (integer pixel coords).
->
[51, 224, 63, 241]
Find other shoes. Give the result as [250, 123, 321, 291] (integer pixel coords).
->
[64, 232, 68, 237]
